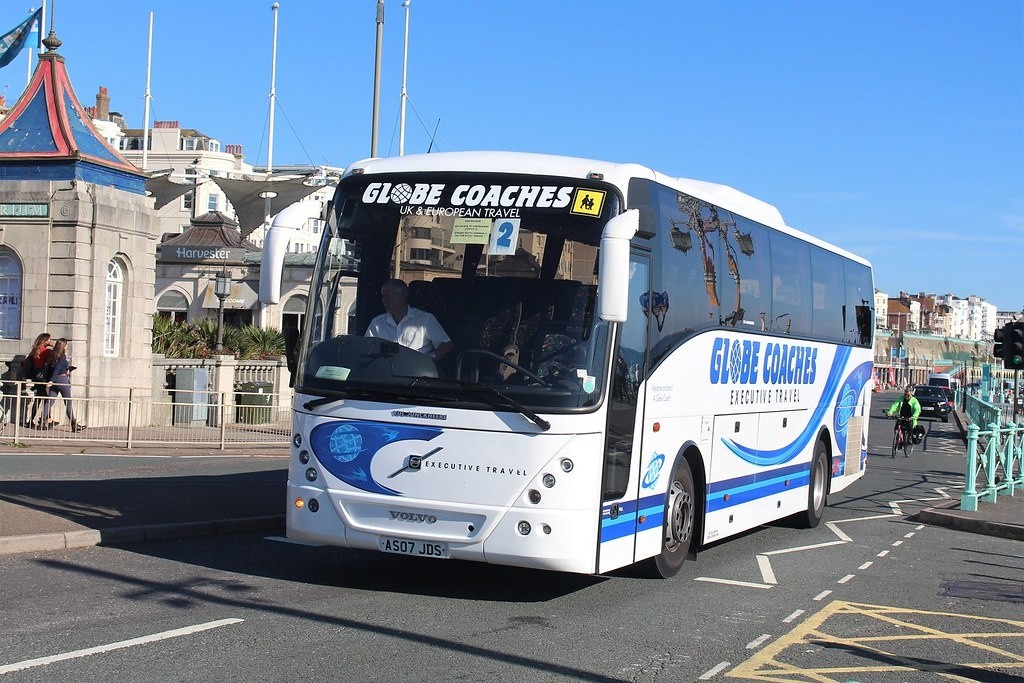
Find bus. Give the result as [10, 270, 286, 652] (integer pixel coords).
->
[284, 150, 876, 579]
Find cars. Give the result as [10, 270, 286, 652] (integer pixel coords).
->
[912, 373, 961, 423]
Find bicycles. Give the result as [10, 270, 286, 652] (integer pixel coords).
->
[886, 414, 915, 459]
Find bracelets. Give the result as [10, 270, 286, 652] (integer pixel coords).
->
[430, 351, 440, 360]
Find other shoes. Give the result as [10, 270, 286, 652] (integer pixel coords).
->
[27, 420, 36, 430]
[37, 421, 47, 430]
[48, 421, 59, 430]
[72, 422, 86, 433]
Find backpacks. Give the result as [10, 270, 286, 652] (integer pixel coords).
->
[19, 353, 35, 379]
[36, 363, 52, 383]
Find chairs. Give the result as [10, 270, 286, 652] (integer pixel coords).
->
[407, 276, 596, 383]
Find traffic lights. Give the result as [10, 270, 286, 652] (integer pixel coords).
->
[993, 328, 1005, 357]
[1010, 329, 1023, 366]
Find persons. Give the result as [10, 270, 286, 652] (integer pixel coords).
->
[27, 332, 59, 429]
[364, 278, 453, 359]
[885, 385, 922, 449]
[38, 338, 87, 433]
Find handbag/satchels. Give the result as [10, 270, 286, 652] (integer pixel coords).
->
[911, 425, 925, 445]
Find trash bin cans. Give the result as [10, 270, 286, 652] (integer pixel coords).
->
[240, 381, 273, 425]
